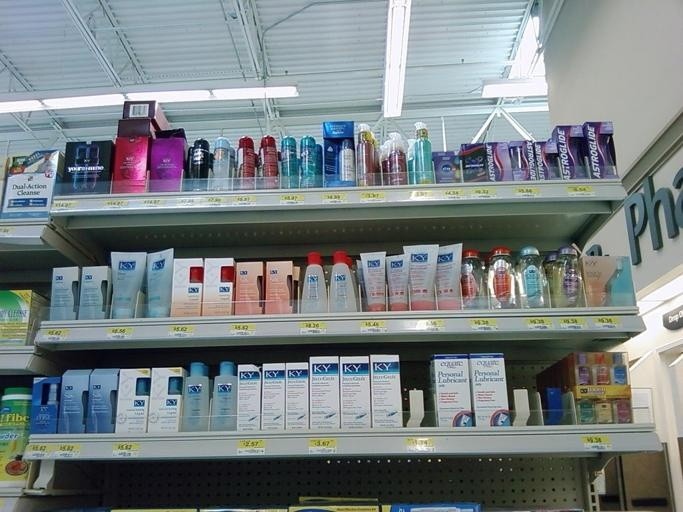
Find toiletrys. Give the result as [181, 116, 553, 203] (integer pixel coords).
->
[61, 101, 619, 199]
[28, 349, 635, 437]
[48, 242, 638, 322]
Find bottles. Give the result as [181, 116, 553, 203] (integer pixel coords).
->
[461, 244, 585, 310]
[298, 249, 360, 314]
[180, 360, 237, 432]
[188, 121, 436, 191]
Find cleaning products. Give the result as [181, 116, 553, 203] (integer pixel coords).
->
[299, 249, 357, 315]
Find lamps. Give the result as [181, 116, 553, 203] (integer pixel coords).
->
[0, 79, 298, 116]
[380, 2, 547, 121]
